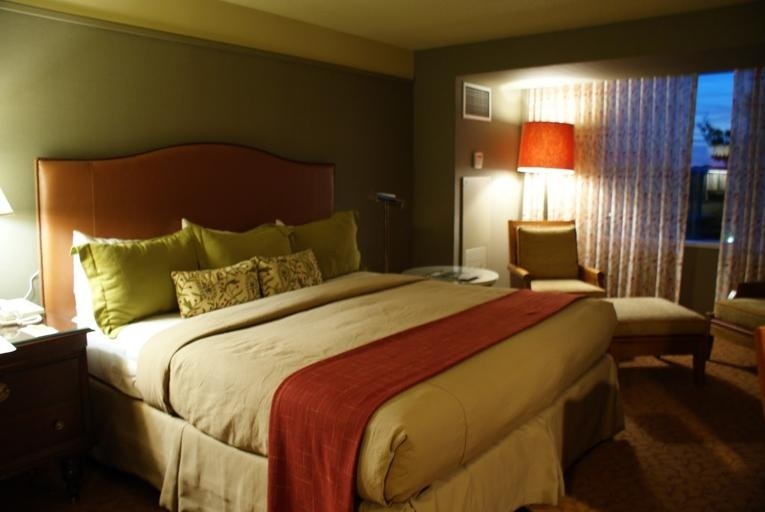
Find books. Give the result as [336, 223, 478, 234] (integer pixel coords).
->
[432, 271, 478, 281]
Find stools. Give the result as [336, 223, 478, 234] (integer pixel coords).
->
[599, 296, 714, 386]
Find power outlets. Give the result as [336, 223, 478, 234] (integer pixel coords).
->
[517, 120, 576, 221]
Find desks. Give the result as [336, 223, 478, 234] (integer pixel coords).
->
[402, 265, 499, 287]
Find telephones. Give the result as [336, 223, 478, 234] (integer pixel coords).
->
[0, 298, 45, 326]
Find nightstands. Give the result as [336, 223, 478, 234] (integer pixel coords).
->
[0, 311, 98, 506]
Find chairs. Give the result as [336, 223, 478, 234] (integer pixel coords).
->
[508, 219, 610, 296]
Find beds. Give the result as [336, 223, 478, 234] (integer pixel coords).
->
[32, 143, 617, 511]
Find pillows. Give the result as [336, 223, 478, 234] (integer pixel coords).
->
[67, 209, 363, 344]
